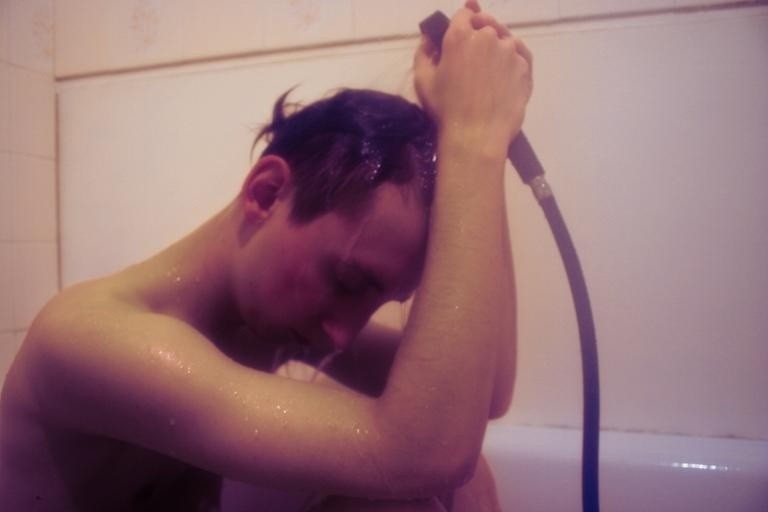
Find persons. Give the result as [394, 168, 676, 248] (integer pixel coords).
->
[1, 2, 535, 512]
